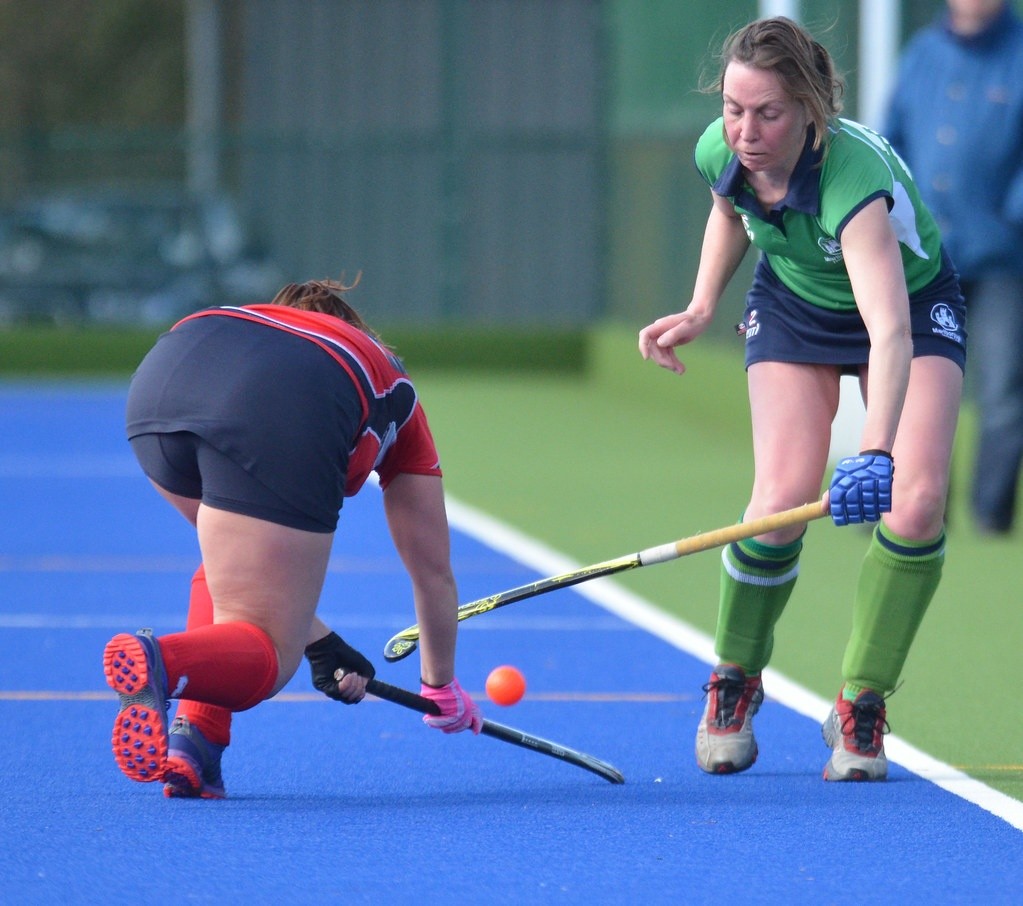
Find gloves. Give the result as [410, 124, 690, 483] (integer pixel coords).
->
[418, 678, 483, 734]
[830, 451, 895, 526]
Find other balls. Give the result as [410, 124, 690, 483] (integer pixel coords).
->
[485, 666, 527, 707]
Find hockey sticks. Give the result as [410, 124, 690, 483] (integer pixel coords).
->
[383, 499, 831, 664]
[333, 667, 625, 785]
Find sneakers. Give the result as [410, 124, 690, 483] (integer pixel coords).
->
[160, 718, 226, 799]
[820, 681, 905, 781]
[103, 628, 169, 782]
[696, 664, 765, 771]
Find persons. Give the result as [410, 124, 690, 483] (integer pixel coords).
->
[104, 269, 482, 799]
[639, 17, 972, 778]
[877, 1, 1023, 534]
